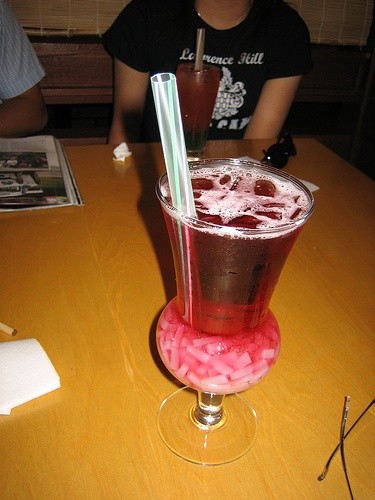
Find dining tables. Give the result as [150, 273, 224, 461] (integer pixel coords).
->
[0, 136, 375, 500]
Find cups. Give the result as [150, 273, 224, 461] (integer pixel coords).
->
[176, 63, 220, 157]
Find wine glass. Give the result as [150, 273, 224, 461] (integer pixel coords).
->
[156, 158, 316, 465]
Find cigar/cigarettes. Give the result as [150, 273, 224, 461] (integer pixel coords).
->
[0, 320, 17, 337]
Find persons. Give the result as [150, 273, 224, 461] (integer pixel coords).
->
[0, 0, 48, 139]
[100, 0, 310, 146]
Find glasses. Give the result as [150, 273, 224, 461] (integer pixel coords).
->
[261, 135, 296, 169]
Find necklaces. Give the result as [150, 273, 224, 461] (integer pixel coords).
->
[194, 1, 254, 30]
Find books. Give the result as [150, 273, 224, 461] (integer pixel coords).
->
[0, 135, 86, 212]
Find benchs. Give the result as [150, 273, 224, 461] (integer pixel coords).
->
[32, 43, 375, 165]
